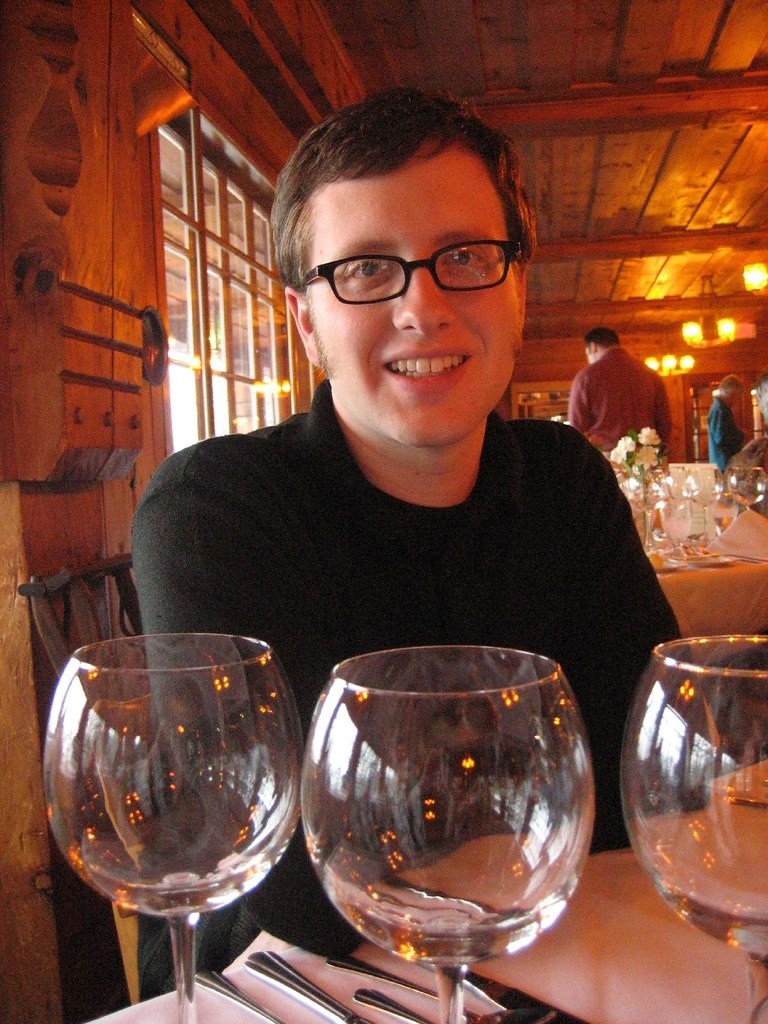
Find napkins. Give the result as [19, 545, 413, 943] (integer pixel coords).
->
[704, 508, 768, 563]
[88, 929, 504, 1024]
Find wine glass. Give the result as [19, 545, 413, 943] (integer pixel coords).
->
[43, 632, 305, 1024]
[620, 633, 768, 1024]
[301, 645, 597, 1024]
[626, 466, 766, 555]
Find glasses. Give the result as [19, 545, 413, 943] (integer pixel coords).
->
[295, 239, 522, 305]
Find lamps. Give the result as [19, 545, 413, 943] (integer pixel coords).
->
[681, 273, 736, 349]
[647, 354, 696, 377]
[743, 262, 768, 296]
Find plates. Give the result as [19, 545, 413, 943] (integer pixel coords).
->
[668, 556, 737, 568]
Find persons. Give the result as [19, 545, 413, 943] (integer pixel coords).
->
[707, 374, 747, 474]
[131, 84, 714, 1005]
[567, 328, 673, 457]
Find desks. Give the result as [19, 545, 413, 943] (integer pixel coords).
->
[659, 562, 768, 667]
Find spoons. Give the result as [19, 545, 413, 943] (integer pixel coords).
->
[326, 954, 557, 1024]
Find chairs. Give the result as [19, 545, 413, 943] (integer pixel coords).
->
[16, 550, 240, 992]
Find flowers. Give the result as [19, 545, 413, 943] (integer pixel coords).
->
[609, 426, 672, 546]
